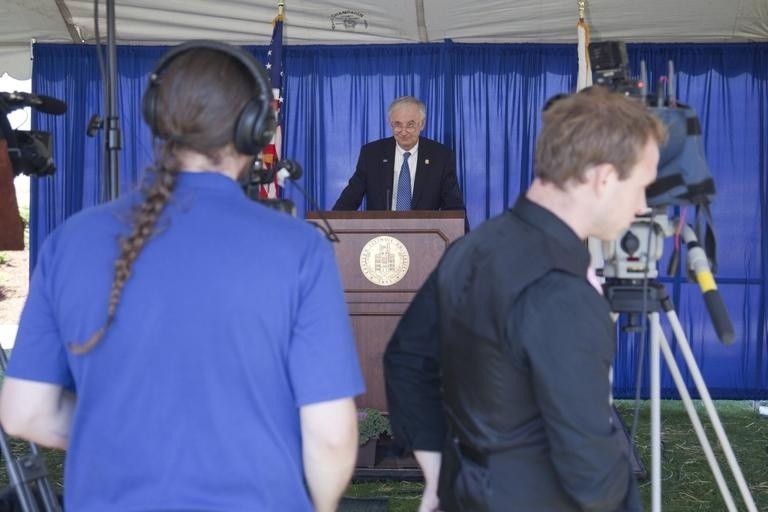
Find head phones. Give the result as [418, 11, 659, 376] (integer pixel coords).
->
[143, 40, 277, 154]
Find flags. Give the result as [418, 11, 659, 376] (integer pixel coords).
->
[575, 17, 607, 296]
[256, 13, 285, 201]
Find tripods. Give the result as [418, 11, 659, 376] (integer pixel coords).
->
[596, 210, 759, 511]
[0, 346, 62, 512]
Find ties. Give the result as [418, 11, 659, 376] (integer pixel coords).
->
[396, 153, 412, 211]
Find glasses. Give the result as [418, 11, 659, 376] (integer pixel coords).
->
[391, 126, 420, 132]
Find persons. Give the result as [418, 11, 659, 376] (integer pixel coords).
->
[332, 93, 470, 238]
[382, 80, 669, 511]
[1, 38, 367, 512]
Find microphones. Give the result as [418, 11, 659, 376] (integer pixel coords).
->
[281, 159, 303, 181]
[385, 184, 391, 210]
[253, 156, 262, 172]
